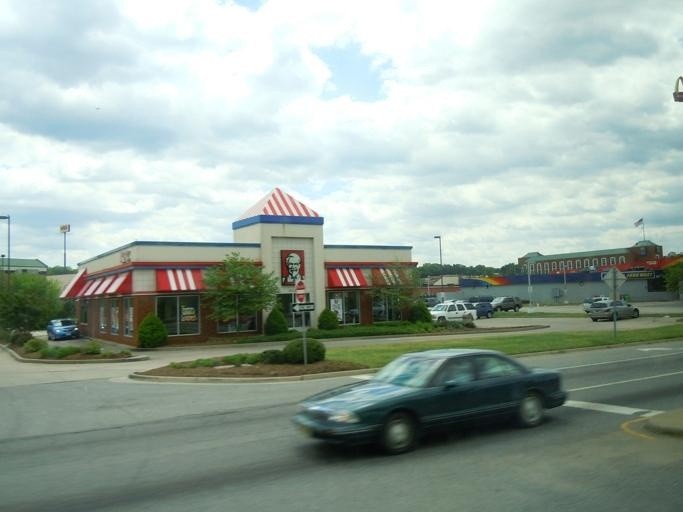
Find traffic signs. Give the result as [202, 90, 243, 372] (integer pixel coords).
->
[290, 302, 314, 312]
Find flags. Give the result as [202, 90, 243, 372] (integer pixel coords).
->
[634, 217, 643, 226]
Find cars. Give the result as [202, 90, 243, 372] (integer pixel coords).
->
[47, 318, 81, 340]
[584, 294, 639, 321]
[291, 346, 566, 456]
[425, 297, 523, 320]
[348, 300, 384, 318]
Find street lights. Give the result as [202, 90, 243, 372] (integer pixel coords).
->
[434, 235, 443, 288]
[0, 215, 10, 277]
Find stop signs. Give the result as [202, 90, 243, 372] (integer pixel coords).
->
[294, 280, 305, 302]
